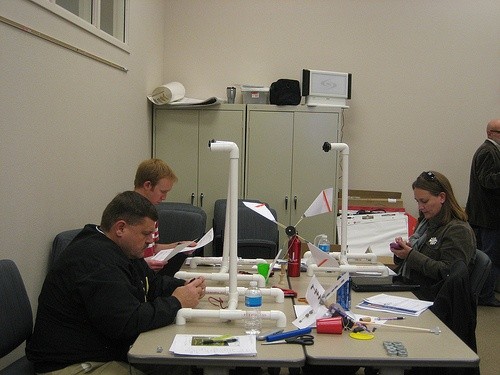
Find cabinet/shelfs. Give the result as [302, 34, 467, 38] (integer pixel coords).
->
[153, 104, 246, 257]
[245, 103, 341, 258]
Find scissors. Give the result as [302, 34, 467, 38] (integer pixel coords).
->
[261, 335, 315, 346]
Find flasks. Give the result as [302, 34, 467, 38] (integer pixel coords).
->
[286, 232, 302, 277]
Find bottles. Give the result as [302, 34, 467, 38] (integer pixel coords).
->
[318, 235, 330, 255]
[244, 281, 263, 335]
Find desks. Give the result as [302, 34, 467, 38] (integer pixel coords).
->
[127, 258, 306, 375]
[287, 259, 480, 375]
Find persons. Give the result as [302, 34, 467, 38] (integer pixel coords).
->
[464, 118, 500, 306]
[133, 158, 197, 275]
[390, 171, 476, 322]
[26, 191, 206, 375]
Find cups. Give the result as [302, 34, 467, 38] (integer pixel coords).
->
[257, 263, 269, 282]
[227, 87, 236, 104]
[316, 317, 343, 335]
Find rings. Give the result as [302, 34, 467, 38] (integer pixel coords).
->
[202, 288, 204, 292]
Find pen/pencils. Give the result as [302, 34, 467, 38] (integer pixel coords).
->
[266, 327, 311, 342]
[204, 339, 237, 344]
[374, 316, 405, 320]
[258, 328, 284, 341]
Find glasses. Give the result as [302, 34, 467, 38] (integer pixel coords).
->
[423, 172, 444, 192]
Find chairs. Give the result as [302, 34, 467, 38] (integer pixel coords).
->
[1, 259, 33, 375]
[155, 202, 207, 256]
[213, 199, 280, 259]
